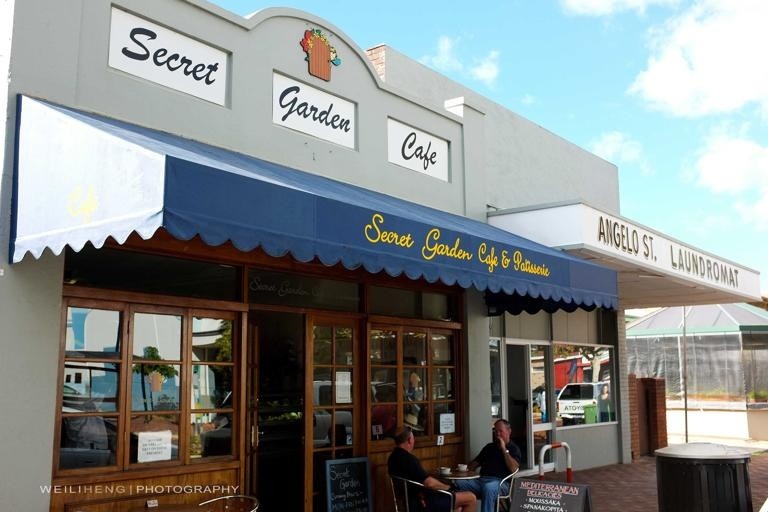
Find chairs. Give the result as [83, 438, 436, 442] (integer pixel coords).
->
[390, 475, 455, 511]
[496, 468, 519, 511]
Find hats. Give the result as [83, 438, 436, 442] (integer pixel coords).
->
[404, 415, 424, 431]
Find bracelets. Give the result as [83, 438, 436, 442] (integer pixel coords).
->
[503, 449, 509, 455]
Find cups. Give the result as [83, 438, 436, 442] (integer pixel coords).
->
[440, 467, 450, 474]
[457, 464, 467, 472]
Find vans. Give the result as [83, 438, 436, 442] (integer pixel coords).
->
[557, 381, 611, 426]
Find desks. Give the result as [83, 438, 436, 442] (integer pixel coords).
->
[443, 471, 481, 492]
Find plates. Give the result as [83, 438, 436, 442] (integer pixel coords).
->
[437, 472, 452, 476]
[456, 468, 469, 472]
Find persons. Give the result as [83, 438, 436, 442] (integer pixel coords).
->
[452, 418, 523, 512]
[535, 383, 548, 423]
[597, 383, 611, 423]
[370, 391, 398, 440]
[386, 429, 477, 512]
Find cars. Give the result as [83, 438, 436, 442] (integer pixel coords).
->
[312, 379, 405, 445]
